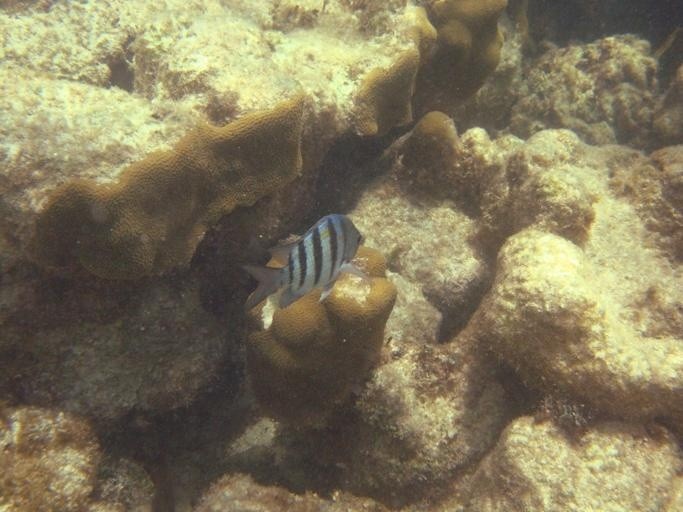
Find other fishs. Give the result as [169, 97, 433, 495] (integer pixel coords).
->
[241, 213, 361, 312]
